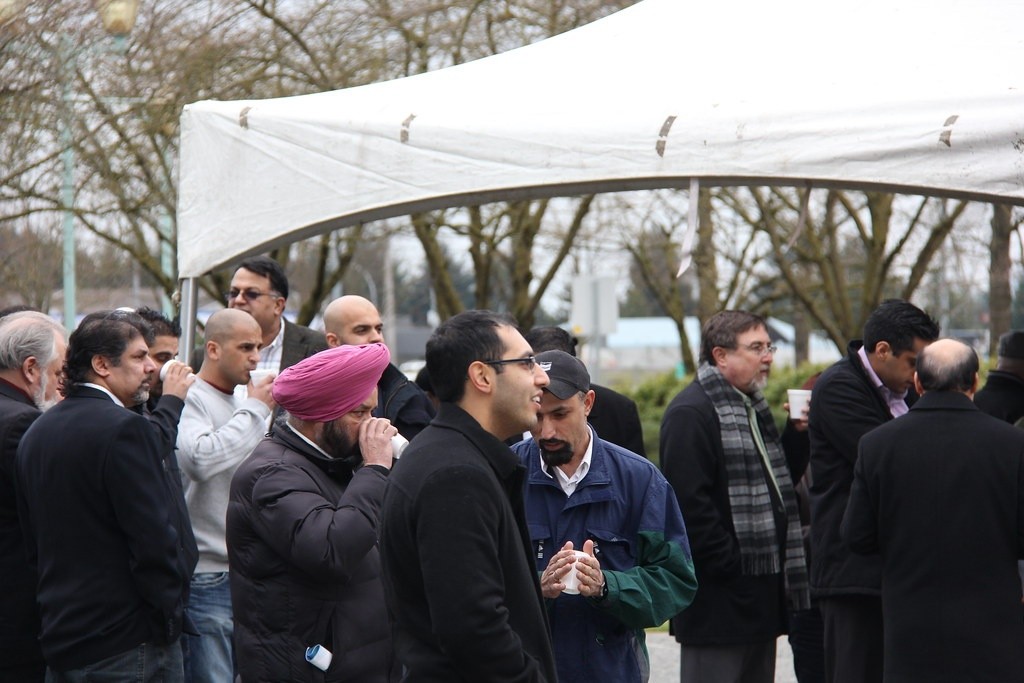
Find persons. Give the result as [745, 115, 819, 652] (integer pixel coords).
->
[659, 310, 810, 683]
[972, 330, 1024, 426]
[11, 309, 188, 683]
[508, 349, 699, 683]
[324, 295, 437, 467]
[126, 306, 202, 638]
[414, 326, 647, 459]
[194, 255, 331, 418]
[379, 309, 558, 683]
[0, 306, 67, 683]
[174, 308, 280, 683]
[788, 298, 940, 683]
[226, 343, 398, 683]
[840, 339, 1024, 683]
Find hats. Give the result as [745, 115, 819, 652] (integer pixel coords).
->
[272, 343, 390, 421]
[999, 331, 1023, 358]
[537, 349, 591, 399]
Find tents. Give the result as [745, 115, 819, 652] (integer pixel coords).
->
[176, 0, 1024, 366]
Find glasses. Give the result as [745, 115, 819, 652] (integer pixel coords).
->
[738, 345, 777, 359]
[223, 286, 279, 302]
[483, 356, 536, 371]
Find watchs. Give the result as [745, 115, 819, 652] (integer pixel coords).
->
[592, 576, 608, 602]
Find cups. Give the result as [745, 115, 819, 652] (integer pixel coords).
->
[557, 549, 591, 594]
[787, 389, 811, 420]
[159, 360, 196, 389]
[370, 417, 409, 459]
[250, 367, 279, 388]
